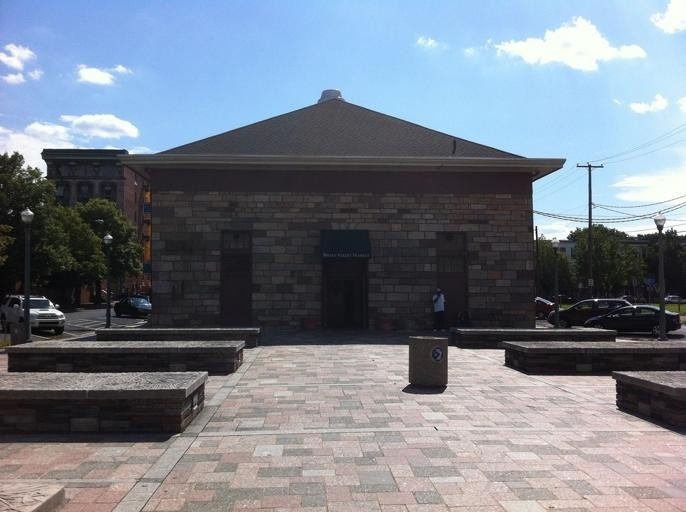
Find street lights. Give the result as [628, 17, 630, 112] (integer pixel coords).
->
[654, 210, 668, 342]
[104, 231, 113, 328]
[21, 207, 36, 341]
[85, 218, 105, 227]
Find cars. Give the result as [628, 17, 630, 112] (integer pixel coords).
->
[535, 292, 686, 335]
[95, 287, 150, 318]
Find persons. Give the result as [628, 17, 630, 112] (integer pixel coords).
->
[432, 287, 446, 332]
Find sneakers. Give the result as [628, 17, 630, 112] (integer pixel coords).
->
[433, 329, 445, 332]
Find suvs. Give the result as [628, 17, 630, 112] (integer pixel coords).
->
[0, 293, 66, 335]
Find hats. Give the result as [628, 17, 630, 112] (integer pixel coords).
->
[437, 288, 440, 291]
